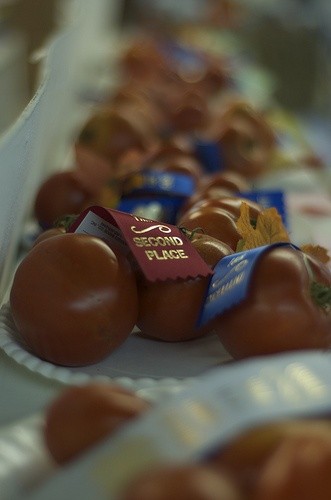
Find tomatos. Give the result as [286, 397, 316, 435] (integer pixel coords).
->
[11, 38, 331, 499]
